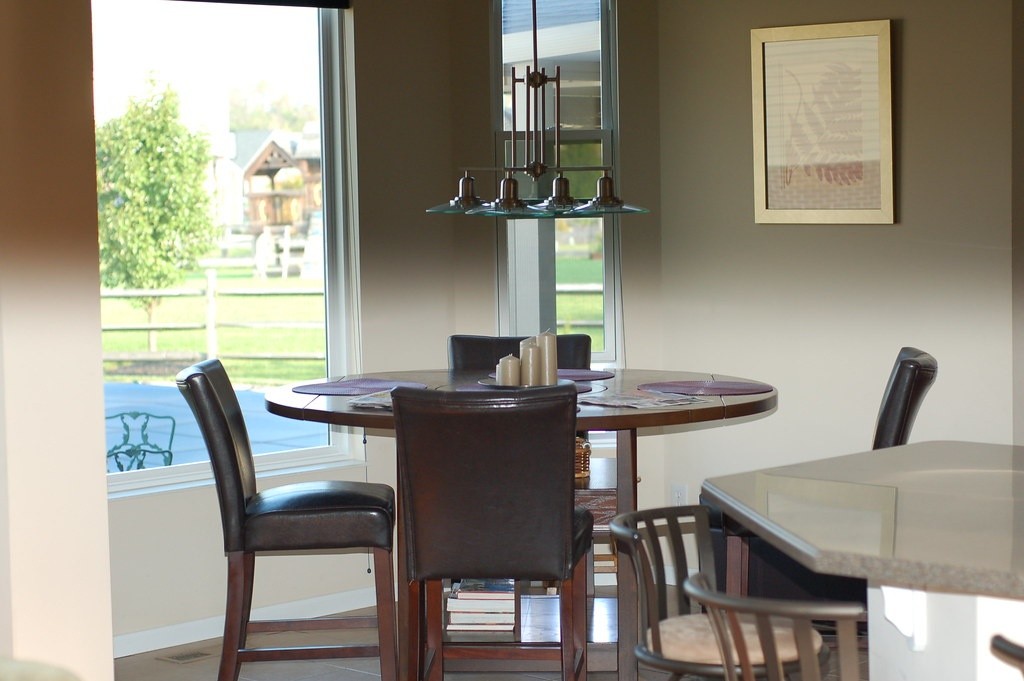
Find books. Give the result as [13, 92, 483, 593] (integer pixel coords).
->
[349, 387, 392, 408]
[445, 579, 515, 630]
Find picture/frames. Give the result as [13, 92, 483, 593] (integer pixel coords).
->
[747, 19, 897, 224]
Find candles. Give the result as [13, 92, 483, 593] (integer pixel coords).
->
[494, 328, 557, 386]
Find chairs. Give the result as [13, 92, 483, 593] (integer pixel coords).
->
[699, 348, 937, 647]
[607, 502, 867, 681]
[172, 360, 399, 681]
[446, 332, 591, 591]
[385, 382, 593, 681]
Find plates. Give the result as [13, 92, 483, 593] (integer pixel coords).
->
[479, 378, 575, 389]
[488, 372, 496, 378]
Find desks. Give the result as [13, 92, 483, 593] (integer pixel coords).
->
[701, 440, 1023, 681]
[266, 367, 778, 679]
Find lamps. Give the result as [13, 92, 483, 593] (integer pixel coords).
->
[425, 0, 650, 217]
